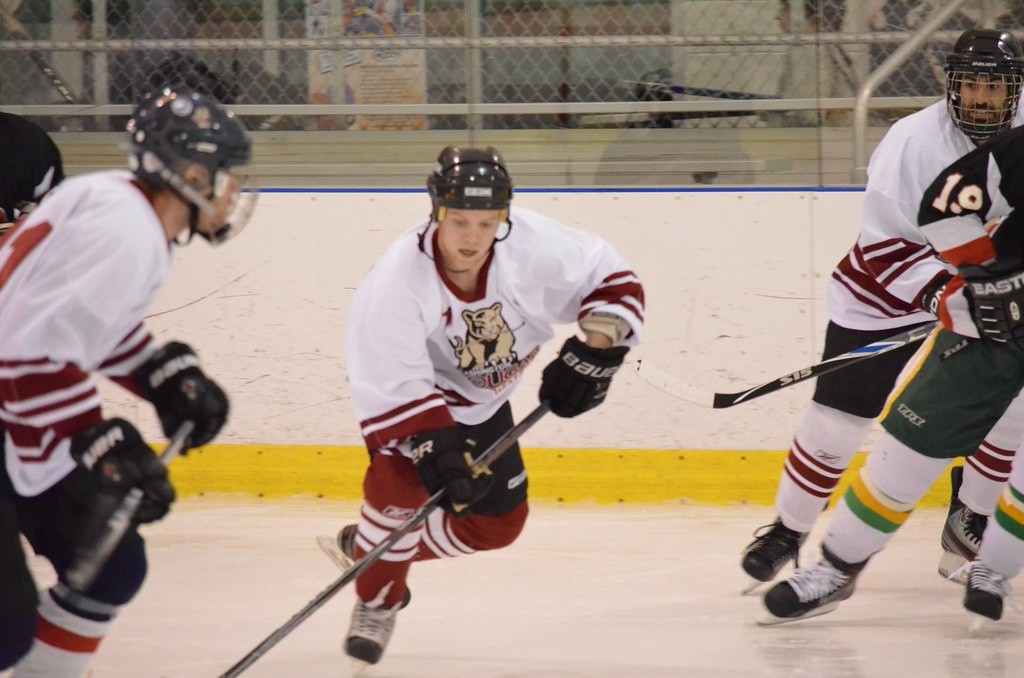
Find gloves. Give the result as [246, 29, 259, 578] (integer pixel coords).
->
[133, 341, 228, 455]
[938, 263, 1024, 343]
[538, 336, 630, 418]
[70, 417, 175, 523]
[410, 427, 494, 514]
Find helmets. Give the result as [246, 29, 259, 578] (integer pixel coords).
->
[942, 28, 1024, 139]
[428, 146, 512, 225]
[126, 78, 256, 248]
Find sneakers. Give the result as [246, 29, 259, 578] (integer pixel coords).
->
[318, 524, 411, 610]
[338, 598, 395, 675]
[939, 465, 990, 584]
[946, 556, 1024, 635]
[740, 517, 810, 584]
[756, 559, 856, 625]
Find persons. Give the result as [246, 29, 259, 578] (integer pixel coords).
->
[337, 145, 646, 665]
[741, 28, 1024, 620]
[0, 86, 253, 678]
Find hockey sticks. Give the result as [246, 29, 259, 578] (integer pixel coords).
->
[59, 416, 199, 598]
[639, 321, 937, 410]
[216, 399, 555, 678]
